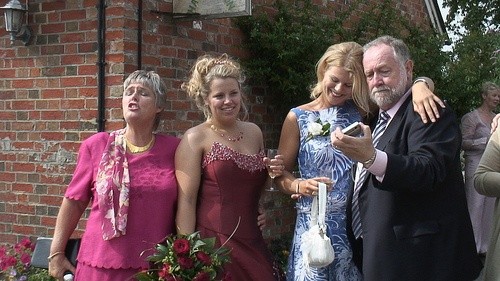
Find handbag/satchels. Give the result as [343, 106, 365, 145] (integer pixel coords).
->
[300, 182, 336, 273]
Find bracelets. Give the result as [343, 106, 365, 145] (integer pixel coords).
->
[414, 79, 429, 88]
[291, 178, 305, 199]
[363, 150, 376, 168]
[48, 251, 65, 261]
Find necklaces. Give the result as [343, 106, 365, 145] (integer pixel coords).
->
[207, 118, 244, 142]
[125, 139, 154, 153]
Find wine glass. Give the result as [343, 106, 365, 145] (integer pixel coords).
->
[320, 167, 338, 216]
[266, 149, 281, 192]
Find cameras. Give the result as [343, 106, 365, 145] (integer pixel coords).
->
[341, 121, 362, 137]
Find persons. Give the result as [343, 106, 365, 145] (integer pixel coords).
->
[461, 82, 500, 256]
[174, 55, 286, 281]
[490, 113, 500, 135]
[331, 35, 483, 281]
[47, 70, 182, 281]
[274, 41, 445, 281]
[474, 114, 500, 281]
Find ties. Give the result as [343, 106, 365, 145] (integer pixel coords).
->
[351, 111, 391, 240]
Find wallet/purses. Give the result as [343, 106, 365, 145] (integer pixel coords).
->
[31, 237, 81, 270]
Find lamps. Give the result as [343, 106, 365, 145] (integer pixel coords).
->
[0, 0, 33, 46]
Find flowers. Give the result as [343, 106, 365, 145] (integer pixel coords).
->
[303, 118, 331, 142]
[133, 225, 235, 281]
